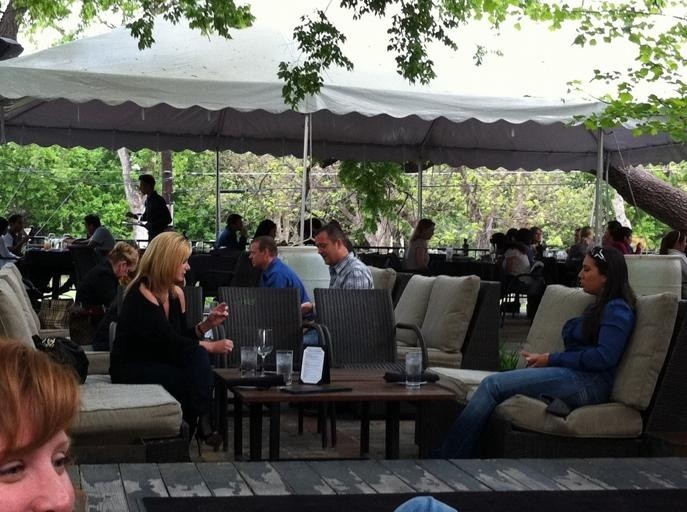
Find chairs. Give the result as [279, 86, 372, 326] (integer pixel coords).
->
[218, 286, 327, 451]
[313, 287, 431, 446]
[0, 260, 183, 463]
[387, 253, 584, 327]
[109, 286, 220, 366]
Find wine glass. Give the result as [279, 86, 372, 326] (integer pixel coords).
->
[254, 326, 276, 377]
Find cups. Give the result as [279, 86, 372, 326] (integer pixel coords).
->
[240, 345, 256, 376]
[275, 348, 294, 385]
[405, 350, 425, 389]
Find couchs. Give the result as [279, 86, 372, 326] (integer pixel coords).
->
[328, 266, 501, 371]
[424, 285, 687, 457]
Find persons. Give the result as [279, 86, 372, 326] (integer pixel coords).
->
[568, 221, 643, 258]
[60, 174, 234, 458]
[249, 237, 311, 303]
[434, 247, 638, 459]
[297, 218, 356, 255]
[481, 226, 542, 301]
[1, 214, 52, 298]
[218, 213, 277, 287]
[404, 219, 435, 270]
[301, 225, 374, 344]
[1, 342, 79, 512]
[659, 231, 686, 298]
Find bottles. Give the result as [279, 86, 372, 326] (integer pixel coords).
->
[43, 236, 63, 250]
[445, 244, 453, 262]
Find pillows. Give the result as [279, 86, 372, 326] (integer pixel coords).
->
[368, 265, 395, 300]
[417, 275, 481, 352]
[389, 273, 437, 343]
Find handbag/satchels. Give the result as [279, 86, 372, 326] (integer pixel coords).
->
[43, 336, 89, 384]
[68, 302, 104, 345]
[39, 300, 74, 329]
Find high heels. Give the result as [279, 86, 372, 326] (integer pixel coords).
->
[195, 431, 222, 457]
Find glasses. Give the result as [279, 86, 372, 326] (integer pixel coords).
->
[591, 247, 607, 263]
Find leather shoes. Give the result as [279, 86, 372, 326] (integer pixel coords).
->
[547, 397, 569, 416]
